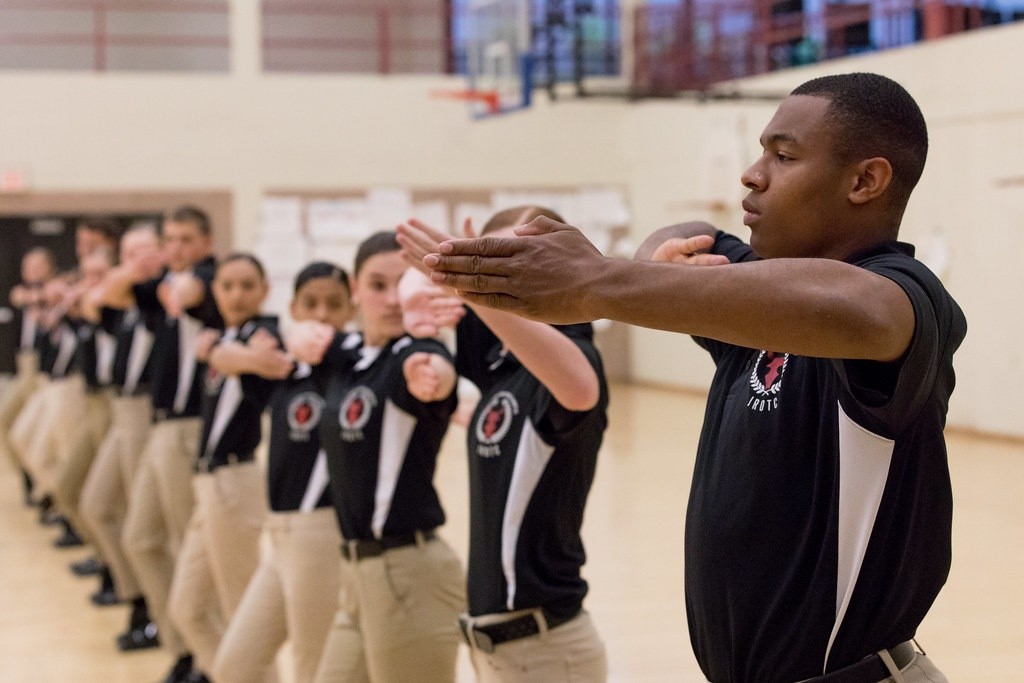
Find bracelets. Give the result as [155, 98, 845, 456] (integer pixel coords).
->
[204, 338, 222, 360]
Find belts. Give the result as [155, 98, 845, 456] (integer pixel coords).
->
[341, 530, 436, 563]
[797, 638, 916, 683]
[457, 603, 581, 654]
[194, 449, 258, 472]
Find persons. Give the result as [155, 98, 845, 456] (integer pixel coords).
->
[425, 73, 970, 683]
[0, 205, 609, 683]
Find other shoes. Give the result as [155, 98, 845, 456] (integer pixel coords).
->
[13, 471, 205, 682]
